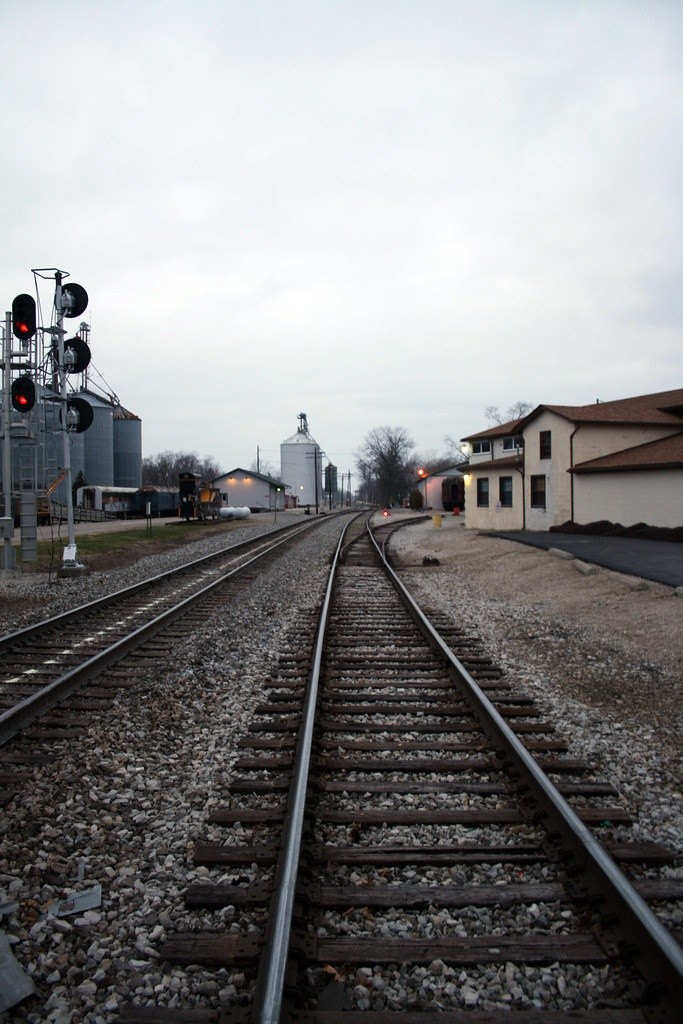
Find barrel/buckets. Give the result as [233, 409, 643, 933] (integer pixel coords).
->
[454, 507, 459, 515]
[432, 514, 441, 528]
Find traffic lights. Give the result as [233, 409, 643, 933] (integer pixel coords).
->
[12, 294, 36, 340]
[11, 377, 35, 412]
